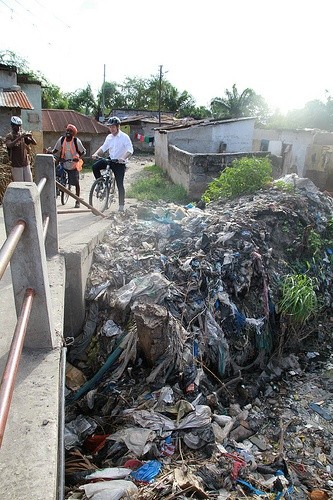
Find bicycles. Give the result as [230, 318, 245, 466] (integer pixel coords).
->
[88, 154, 127, 217]
[48, 150, 79, 205]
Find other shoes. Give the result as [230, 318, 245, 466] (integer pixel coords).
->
[76, 201, 80, 208]
[119, 206, 124, 211]
[95, 185, 103, 192]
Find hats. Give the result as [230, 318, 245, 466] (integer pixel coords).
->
[107, 116, 121, 126]
[11, 115, 22, 126]
[66, 124, 77, 135]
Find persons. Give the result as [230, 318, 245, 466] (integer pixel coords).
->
[5, 115, 37, 183]
[92, 117, 133, 212]
[47, 124, 85, 207]
